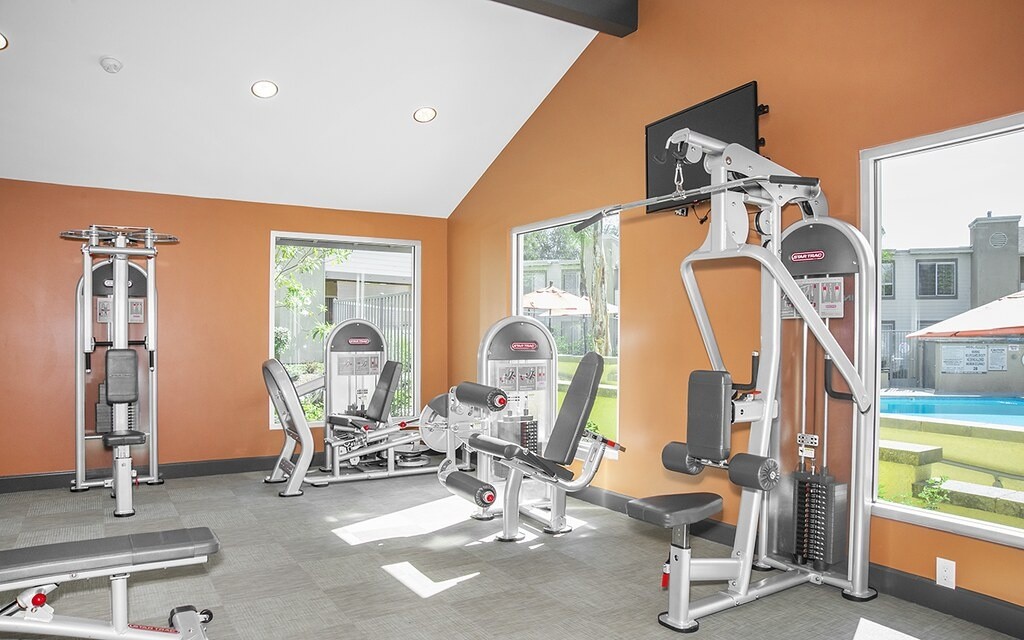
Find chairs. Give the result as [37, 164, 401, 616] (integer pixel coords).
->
[470, 351, 604, 542]
[324, 360, 403, 432]
[626, 370, 733, 633]
[102, 349, 146, 518]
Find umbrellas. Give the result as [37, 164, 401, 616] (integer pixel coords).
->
[906, 290, 1024, 338]
[523, 281, 619, 354]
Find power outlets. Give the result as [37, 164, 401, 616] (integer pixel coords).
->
[935, 556, 956, 590]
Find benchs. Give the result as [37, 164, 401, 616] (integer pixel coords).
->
[0, 526, 220, 633]
[878, 439, 942, 481]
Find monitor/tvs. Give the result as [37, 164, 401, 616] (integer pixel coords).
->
[645, 81, 758, 214]
